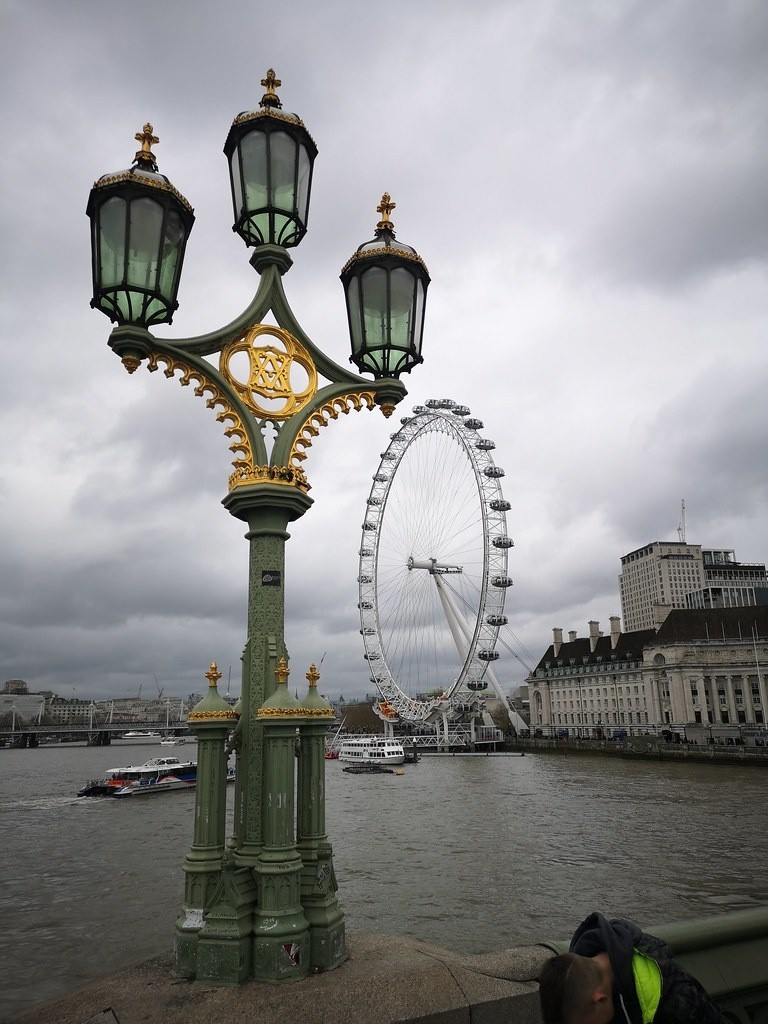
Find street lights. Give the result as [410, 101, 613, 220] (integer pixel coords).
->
[737, 724, 744, 745]
[79, 62, 428, 986]
[708, 724, 715, 746]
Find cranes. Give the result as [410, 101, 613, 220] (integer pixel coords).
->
[151, 671, 165, 700]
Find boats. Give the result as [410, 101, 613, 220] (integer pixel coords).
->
[148, 732, 161, 736]
[323, 747, 339, 759]
[338, 736, 405, 766]
[75, 760, 236, 797]
[125, 730, 150, 736]
[139, 756, 178, 767]
[160, 735, 186, 746]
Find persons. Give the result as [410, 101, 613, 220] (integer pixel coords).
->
[539, 913, 724, 1024]
[112, 771, 123, 780]
